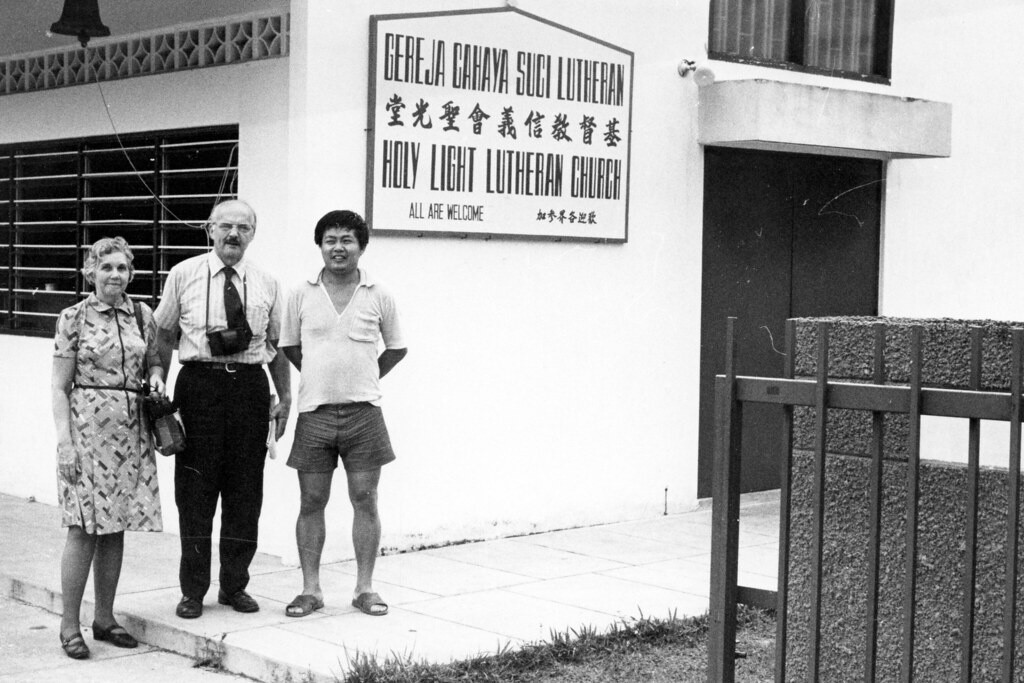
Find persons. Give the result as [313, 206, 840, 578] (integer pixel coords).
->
[143, 200, 291, 618]
[50, 237, 167, 660]
[278, 210, 407, 618]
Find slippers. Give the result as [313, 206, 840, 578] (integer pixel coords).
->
[285, 594, 324, 617]
[352, 591, 389, 615]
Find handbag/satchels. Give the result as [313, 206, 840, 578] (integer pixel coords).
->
[153, 413, 185, 456]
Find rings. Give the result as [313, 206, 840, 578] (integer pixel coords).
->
[60, 470, 64, 472]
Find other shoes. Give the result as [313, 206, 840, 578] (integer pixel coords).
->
[60, 632, 88, 658]
[92, 620, 137, 648]
[176, 595, 203, 618]
[218, 589, 259, 612]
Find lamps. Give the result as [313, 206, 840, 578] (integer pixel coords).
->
[679, 58, 715, 86]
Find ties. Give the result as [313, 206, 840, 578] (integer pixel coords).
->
[221, 267, 253, 354]
